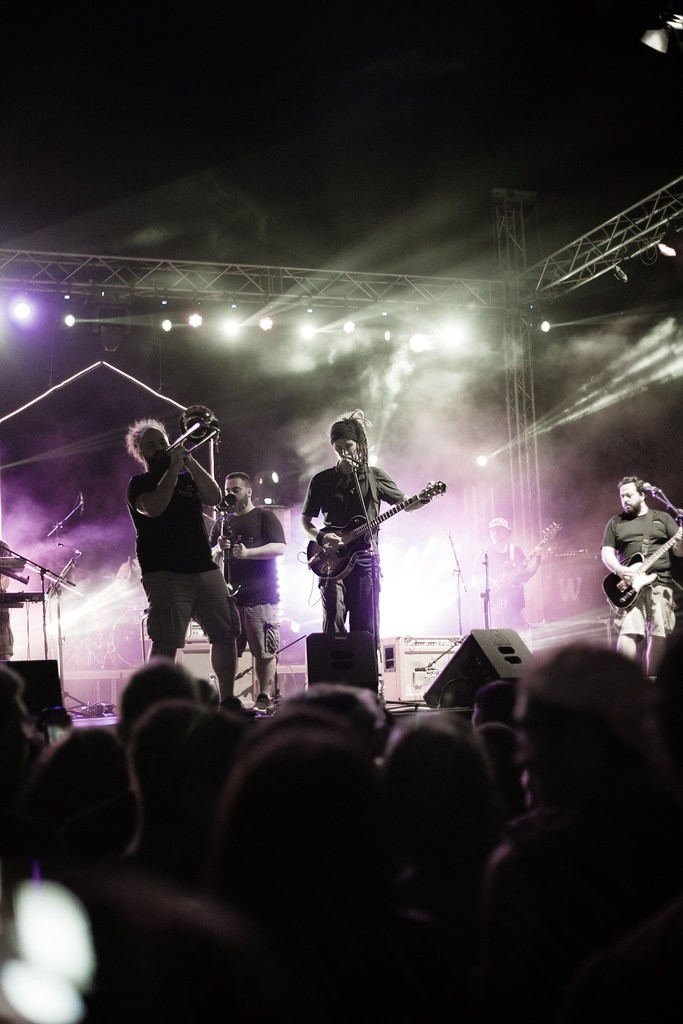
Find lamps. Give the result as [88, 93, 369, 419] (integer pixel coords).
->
[658, 224, 683, 256]
[253, 468, 279, 506]
[611, 265, 628, 283]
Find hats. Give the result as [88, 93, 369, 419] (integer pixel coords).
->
[488, 517, 512, 536]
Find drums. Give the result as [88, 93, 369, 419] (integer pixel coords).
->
[112, 610, 153, 667]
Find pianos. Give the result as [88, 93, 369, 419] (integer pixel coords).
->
[0, 557, 45, 608]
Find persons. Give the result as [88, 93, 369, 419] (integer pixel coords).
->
[124, 418, 261, 720]
[0, 636, 683, 1024]
[208, 472, 287, 713]
[600, 476, 683, 660]
[471, 518, 543, 630]
[300, 409, 438, 690]
[0, 540, 14, 661]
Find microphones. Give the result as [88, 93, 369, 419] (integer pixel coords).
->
[643, 483, 661, 492]
[414, 668, 436, 671]
[345, 455, 360, 467]
[75, 549, 81, 555]
[80, 493, 84, 510]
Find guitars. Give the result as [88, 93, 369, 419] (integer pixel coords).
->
[307, 480, 448, 581]
[601, 528, 683, 611]
[489, 520, 563, 599]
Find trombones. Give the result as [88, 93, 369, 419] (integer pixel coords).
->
[166, 405, 222, 455]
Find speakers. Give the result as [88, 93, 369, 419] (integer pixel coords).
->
[423, 629, 535, 710]
[0, 659, 72, 728]
[176, 643, 260, 710]
[379, 633, 463, 703]
[306, 631, 378, 692]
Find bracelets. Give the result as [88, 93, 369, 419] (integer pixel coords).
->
[316, 531, 325, 546]
[420, 499, 430, 504]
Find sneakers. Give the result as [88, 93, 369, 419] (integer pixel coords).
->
[253, 693, 275, 712]
[220, 696, 257, 718]
